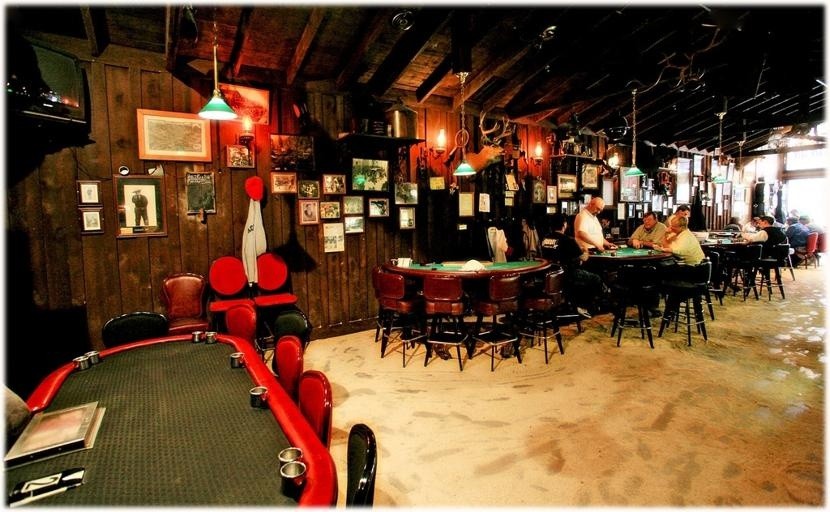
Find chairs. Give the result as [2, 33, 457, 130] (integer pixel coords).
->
[102, 312, 168, 348]
[760, 244, 791, 300]
[799, 232, 819, 269]
[610, 264, 657, 349]
[255, 253, 298, 351]
[514, 269, 565, 364]
[271, 310, 308, 377]
[422, 275, 469, 371]
[161, 273, 209, 334]
[733, 244, 763, 302]
[345, 424, 377, 508]
[723, 224, 741, 231]
[680, 256, 715, 320]
[371, 266, 414, 352]
[225, 304, 256, 340]
[207, 257, 254, 312]
[469, 273, 523, 372]
[274, 335, 303, 406]
[703, 251, 723, 306]
[376, 273, 432, 367]
[296, 370, 332, 450]
[813, 233, 827, 267]
[658, 261, 713, 346]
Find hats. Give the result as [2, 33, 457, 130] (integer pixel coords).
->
[245, 175, 265, 200]
[133, 189, 141, 192]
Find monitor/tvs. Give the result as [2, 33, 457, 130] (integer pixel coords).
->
[6, 31, 88, 128]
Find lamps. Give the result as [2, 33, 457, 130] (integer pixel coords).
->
[198, 44, 237, 121]
[453, 75, 476, 175]
[624, 93, 645, 177]
[711, 116, 728, 184]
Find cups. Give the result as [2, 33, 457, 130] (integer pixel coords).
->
[280, 462, 305, 494]
[205, 332, 216, 343]
[73, 356, 89, 369]
[231, 353, 244, 367]
[191, 331, 203, 343]
[249, 387, 267, 407]
[86, 351, 99, 364]
[279, 448, 303, 465]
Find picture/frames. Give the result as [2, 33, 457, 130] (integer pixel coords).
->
[367, 196, 391, 219]
[323, 223, 344, 253]
[296, 199, 321, 226]
[297, 180, 321, 200]
[269, 171, 298, 194]
[267, 132, 315, 172]
[226, 145, 255, 168]
[399, 207, 415, 230]
[76, 180, 104, 206]
[344, 216, 366, 235]
[184, 172, 216, 214]
[395, 182, 418, 204]
[342, 196, 364, 216]
[77, 208, 105, 234]
[112, 174, 168, 238]
[352, 157, 389, 192]
[137, 109, 212, 163]
[320, 201, 341, 220]
[321, 173, 346, 196]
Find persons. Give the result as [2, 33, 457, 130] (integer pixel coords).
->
[742, 216, 786, 259]
[786, 217, 809, 249]
[655, 216, 705, 293]
[664, 204, 690, 229]
[131, 188, 149, 226]
[725, 209, 823, 233]
[628, 211, 669, 252]
[573, 196, 616, 252]
[543, 213, 600, 318]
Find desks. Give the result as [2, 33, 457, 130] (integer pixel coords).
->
[696, 229, 759, 247]
[582, 246, 673, 264]
[382, 255, 551, 278]
[5, 333, 338, 509]
